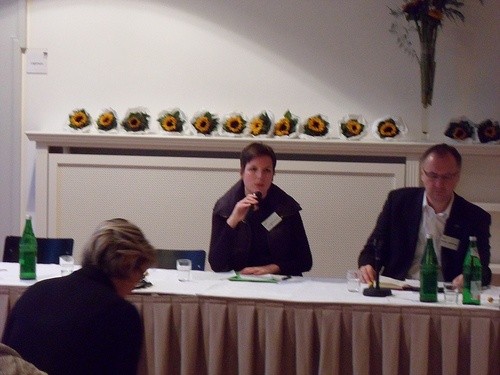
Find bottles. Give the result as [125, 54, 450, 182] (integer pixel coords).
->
[418, 233, 439, 303]
[18, 215, 37, 280]
[462, 236, 482, 306]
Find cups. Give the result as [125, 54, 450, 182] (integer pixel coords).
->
[176, 258, 192, 282]
[346, 269, 361, 292]
[443, 282, 459, 304]
[59, 255, 74, 277]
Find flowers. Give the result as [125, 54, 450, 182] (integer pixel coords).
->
[387, 0, 484, 106]
[96, 111, 118, 131]
[221, 114, 247, 134]
[68, 109, 93, 132]
[444, 121, 475, 141]
[478, 119, 500, 143]
[304, 115, 330, 136]
[248, 112, 272, 135]
[158, 111, 186, 132]
[341, 119, 365, 137]
[121, 111, 151, 132]
[190, 111, 220, 135]
[377, 118, 400, 138]
[273, 110, 298, 136]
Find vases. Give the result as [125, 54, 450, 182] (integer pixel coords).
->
[418, 60, 437, 142]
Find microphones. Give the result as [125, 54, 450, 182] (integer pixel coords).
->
[243, 191, 262, 222]
[372, 233, 386, 268]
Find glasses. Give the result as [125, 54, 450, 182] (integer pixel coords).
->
[130, 265, 148, 290]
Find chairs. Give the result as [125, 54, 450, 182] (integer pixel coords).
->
[3, 236, 74, 264]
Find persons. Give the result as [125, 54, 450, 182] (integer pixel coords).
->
[357, 143, 492, 289]
[2, 216, 157, 375]
[208, 142, 313, 276]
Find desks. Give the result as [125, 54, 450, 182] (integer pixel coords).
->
[0, 261, 500, 375]
